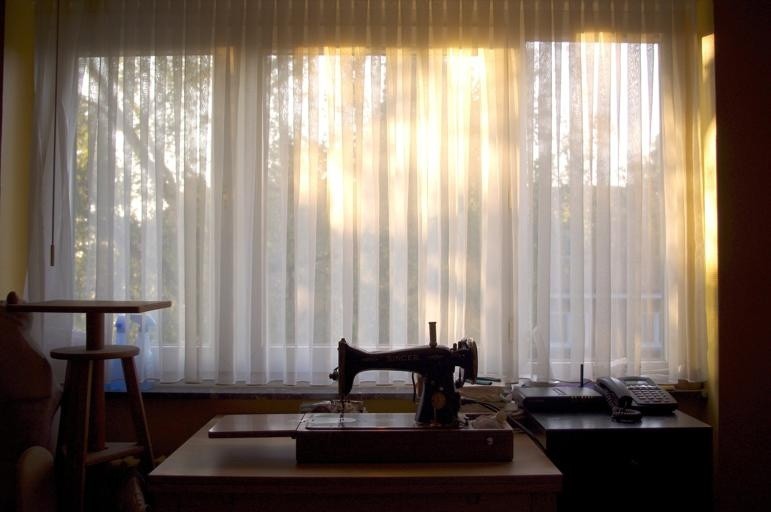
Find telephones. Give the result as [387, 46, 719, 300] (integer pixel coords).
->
[595, 374, 677, 420]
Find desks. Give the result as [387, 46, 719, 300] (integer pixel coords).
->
[8, 299, 172, 511]
[147, 411, 562, 510]
